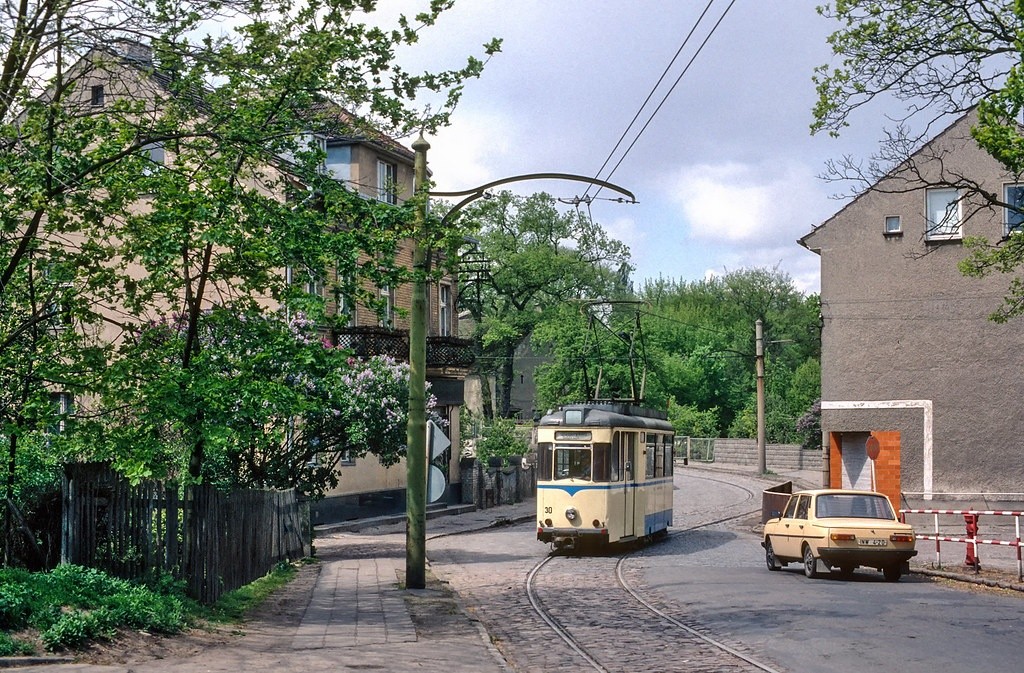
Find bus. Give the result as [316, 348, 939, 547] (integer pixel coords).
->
[534, 296, 677, 554]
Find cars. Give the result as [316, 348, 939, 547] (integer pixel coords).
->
[760, 487, 918, 582]
